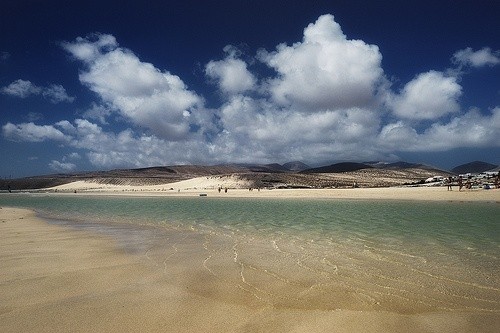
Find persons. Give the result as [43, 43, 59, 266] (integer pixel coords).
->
[458, 176, 463, 192]
[217, 183, 260, 194]
[447, 178, 453, 191]
[354, 181, 355, 187]
